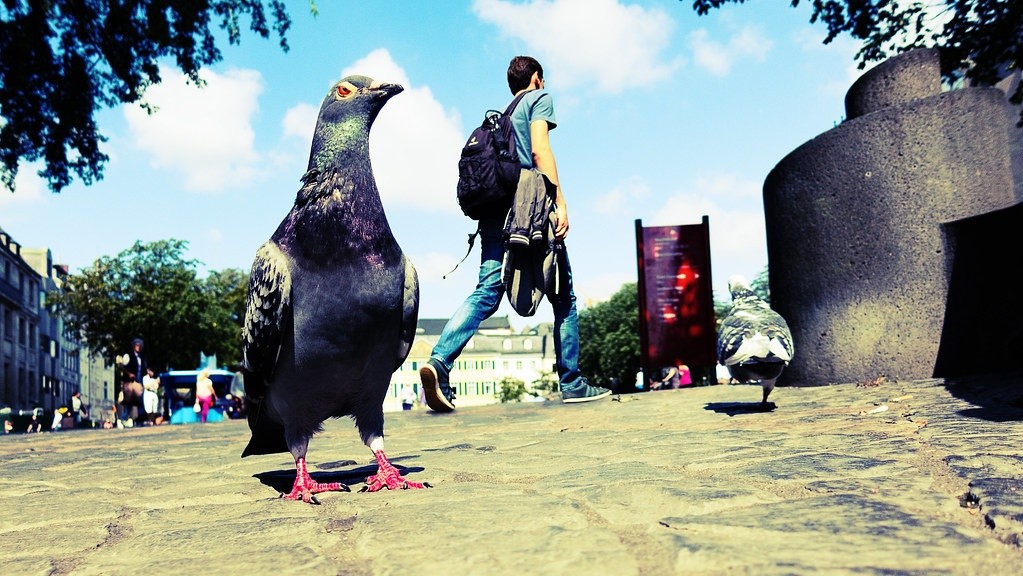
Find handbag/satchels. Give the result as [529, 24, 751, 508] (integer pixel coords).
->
[194, 402, 200, 412]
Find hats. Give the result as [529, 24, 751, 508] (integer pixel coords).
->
[134, 339, 143, 345]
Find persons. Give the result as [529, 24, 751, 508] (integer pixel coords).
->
[68, 391, 87, 427]
[419, 56, 612, 411]
[663, 358, 692, 389]
[196, 369, 218, 423]
[401, 382, 428, 411]
[103, 341, 161, 429]
[230, 370, 246, 418]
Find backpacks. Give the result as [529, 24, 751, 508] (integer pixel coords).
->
[457, 89, 533, 220]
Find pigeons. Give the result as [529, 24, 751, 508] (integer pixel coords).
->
[239, 76, 466, 506]
[715, 271, 797, 416]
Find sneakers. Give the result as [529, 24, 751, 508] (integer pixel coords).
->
[562, 377, 612, 403]
[419, 357, 457, 412]
